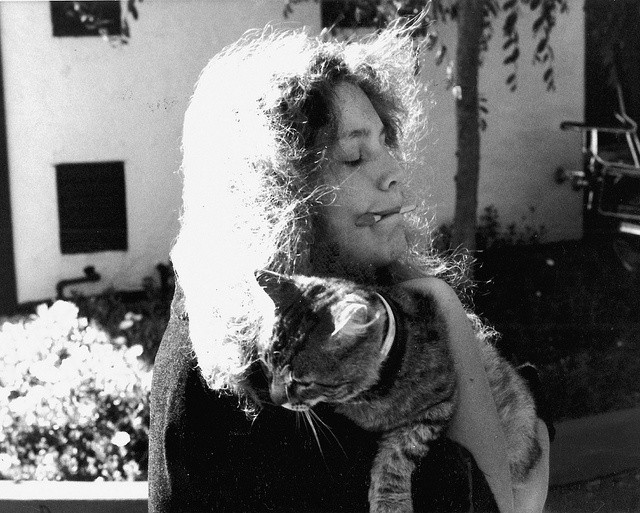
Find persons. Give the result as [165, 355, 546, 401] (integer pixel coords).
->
[148, 11, 558, 512]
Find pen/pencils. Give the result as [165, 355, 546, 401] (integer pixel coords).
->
[355, 205, 420, 225]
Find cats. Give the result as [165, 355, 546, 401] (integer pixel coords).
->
[249, 264, 543, 507]
